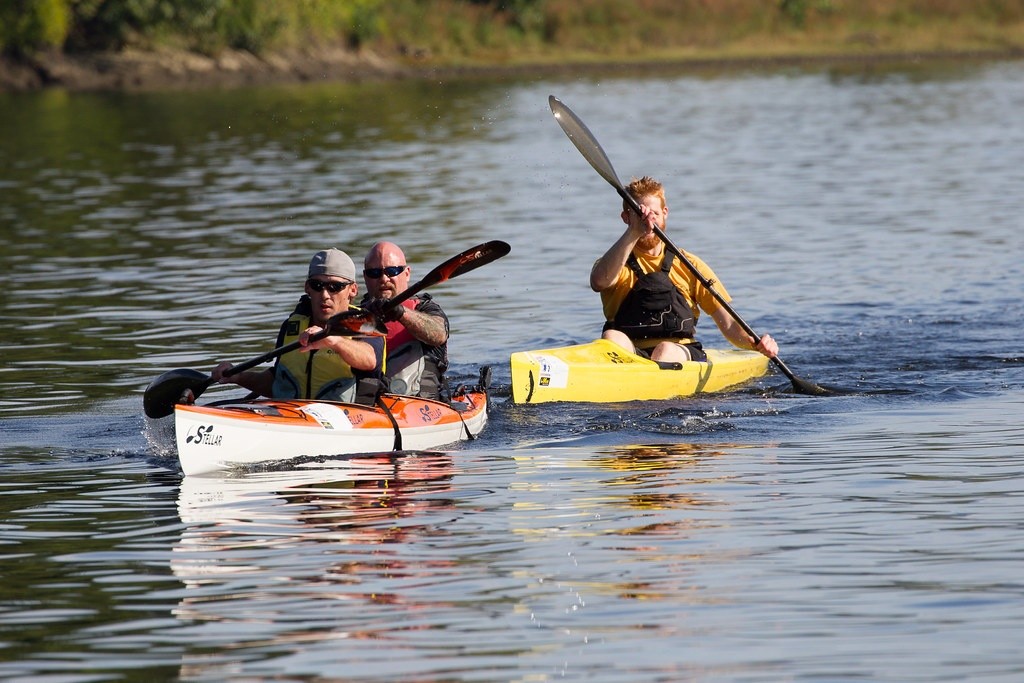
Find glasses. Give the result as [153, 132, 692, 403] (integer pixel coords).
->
[364, 266, 406, 278]
[307, 279, 354, 292]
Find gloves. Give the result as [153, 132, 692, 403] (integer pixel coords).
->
[364, 297, 404, 323]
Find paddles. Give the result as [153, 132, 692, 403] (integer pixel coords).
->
[144, 240, 511, 418]
[547, 94, 823, 391]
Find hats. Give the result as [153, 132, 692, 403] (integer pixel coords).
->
[308, 247, 357, 283]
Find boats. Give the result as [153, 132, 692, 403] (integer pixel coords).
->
[174, 365, 494, 476]
[509, 338, 769, 406]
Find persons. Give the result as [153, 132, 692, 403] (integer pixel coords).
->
[591, 176, 780, 363]
[357, 240, 451, 399]
[211, 247, 385, 403]
